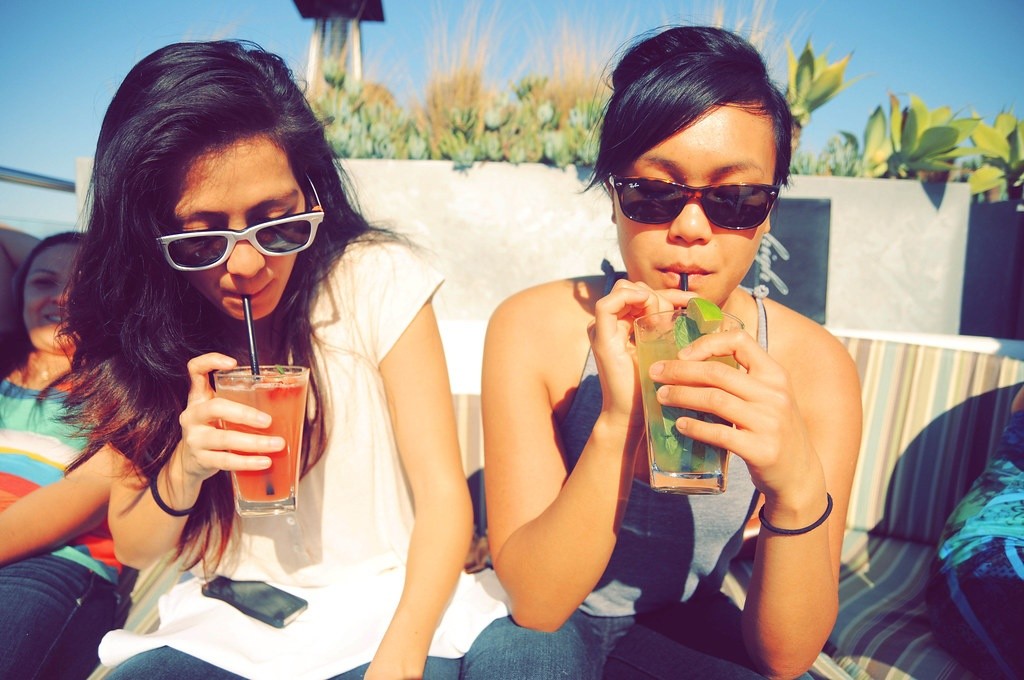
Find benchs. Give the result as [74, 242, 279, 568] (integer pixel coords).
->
[716, 328, 1024, 679]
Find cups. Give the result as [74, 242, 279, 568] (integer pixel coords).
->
[213, 365, 309, 517]
[634, 308, 746, 493]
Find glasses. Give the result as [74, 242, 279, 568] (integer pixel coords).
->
[154, 173, 326, 272]
[612, 173, 780, 230]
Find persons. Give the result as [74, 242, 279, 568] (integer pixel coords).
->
[463, 27, 864, 680]
[43, 41, 510, 680]
[0, 232, 126, 680]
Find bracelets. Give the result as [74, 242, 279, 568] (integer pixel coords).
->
[151, 458, 198, 516]
[758, 492, 833, 534]
